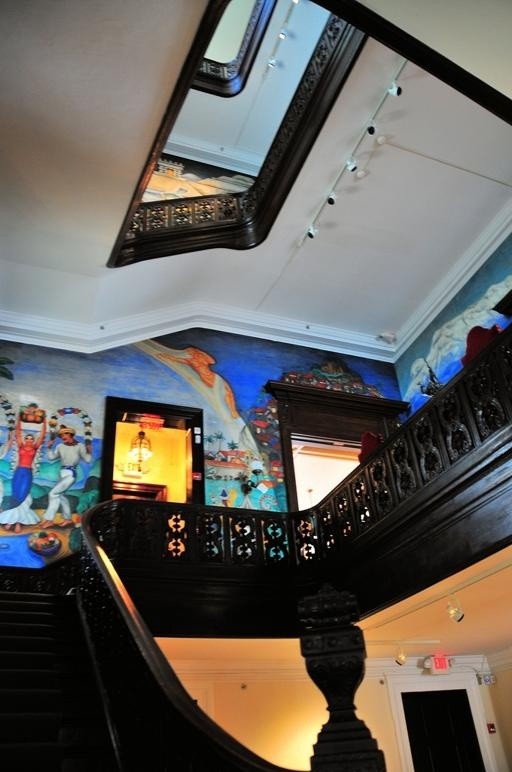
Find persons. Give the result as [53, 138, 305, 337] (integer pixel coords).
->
[0, 414, 46, 534]
[0, 431, 14, 509]
[38, 423, 93, 530]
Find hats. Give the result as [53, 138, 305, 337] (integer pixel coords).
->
[58, 425, 75, 438]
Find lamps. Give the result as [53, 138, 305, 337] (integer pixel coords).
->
[445, 594, 465, 623]
[346, 160, 357, 172]
[267, 58, 279, 69]
[394, 644, 409, 666]
[278, 27, 288, 40]
[385, 81, 402, 96]
[308, 224, 319, 239]
[327, 192, 337, 205]
[366, 121, 376, 136]
[423, 654, 435, 668]
[123, 430, 151, 477]
